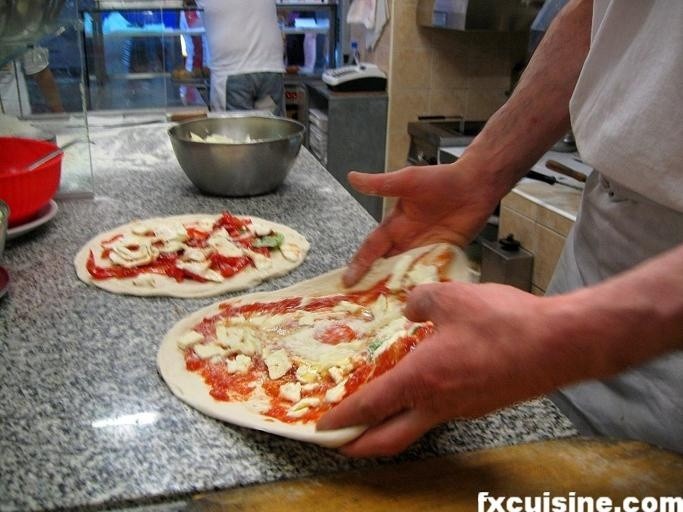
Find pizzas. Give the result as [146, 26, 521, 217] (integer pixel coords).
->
[73, 213, 309, 297]
[156, 244, 470, 447]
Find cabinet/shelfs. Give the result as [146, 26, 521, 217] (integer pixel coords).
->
[91, 0, 336, 122]
[499, 189, 574, 295]
[304, 81, 387, 221]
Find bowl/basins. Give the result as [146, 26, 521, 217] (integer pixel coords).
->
[166, 115, 307, 200]
[0, 136, 63, 225]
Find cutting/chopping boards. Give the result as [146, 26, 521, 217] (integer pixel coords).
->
[187, 436, 682, 511]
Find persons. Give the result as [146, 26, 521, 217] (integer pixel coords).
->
[314, 0, 682, 460]
[177, 12, 209, 107]
[0, 43, 63, 113]
[195, 0, 286, 118]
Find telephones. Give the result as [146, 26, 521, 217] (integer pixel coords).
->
[322, 62, 387, 92]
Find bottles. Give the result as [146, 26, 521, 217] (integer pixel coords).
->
[0, 199, 10, 269]
[348, 40, 359, 67]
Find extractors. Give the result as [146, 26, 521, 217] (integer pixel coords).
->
[416, 1, 537, 33]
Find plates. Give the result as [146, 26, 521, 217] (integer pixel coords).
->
[0, 264, 12, 299]
[7, 196, 57, 242]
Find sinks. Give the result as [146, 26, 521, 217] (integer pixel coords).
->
[406, 119, 486, 147]
